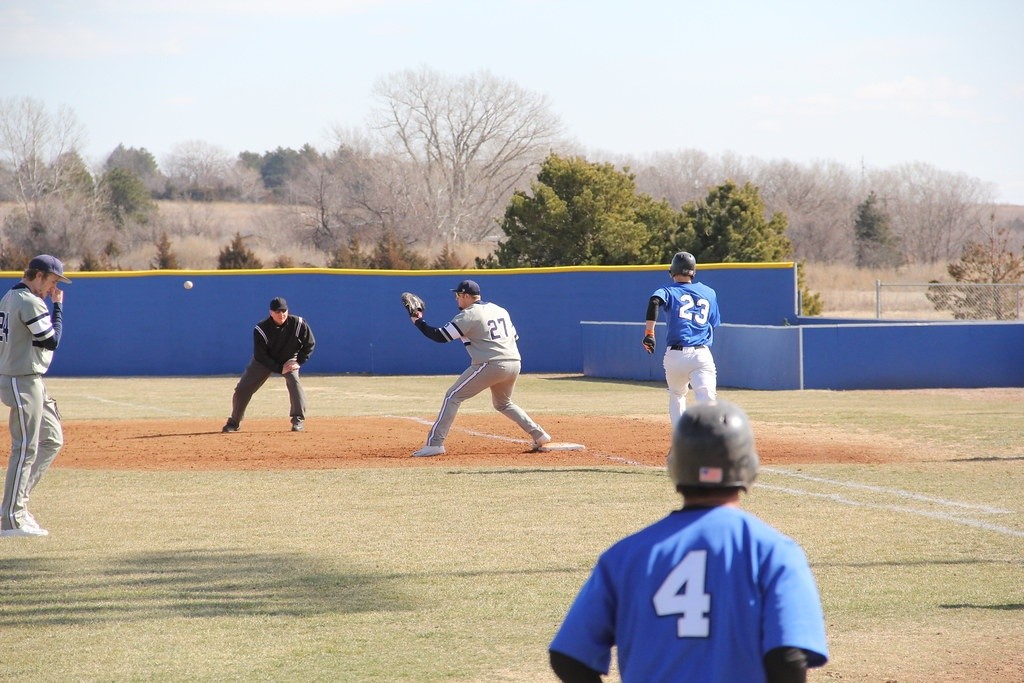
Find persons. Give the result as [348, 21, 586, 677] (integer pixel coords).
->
[0, 253, 72, 537]
[641, 252, 721, 434]
[400, 279, 551, 456]
[548, 400, 831, 683]
[222, 297, 315, 432]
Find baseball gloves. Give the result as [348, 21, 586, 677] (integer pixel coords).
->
[401, 292, 426, 316]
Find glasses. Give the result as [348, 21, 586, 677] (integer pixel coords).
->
[276, 309, 286, 313]
[456, 292, 466, 298]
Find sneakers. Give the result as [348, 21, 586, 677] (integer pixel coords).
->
[0, 505, 38, 528]
[292, 422, 305, 431]
[412, 445, 445, 456]
[0, 526, 49, 536]
[223, 423, 240, 433]
[533, 432, 551, 450]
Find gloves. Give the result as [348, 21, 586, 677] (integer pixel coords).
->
[642, 329, 656, 354]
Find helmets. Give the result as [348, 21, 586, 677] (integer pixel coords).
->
[668, 252, 696, 278]
[668, 400, 759, 494]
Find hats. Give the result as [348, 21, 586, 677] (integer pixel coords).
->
[449, 280, 481, 295]
[269, 298, 289, 310]
[29, 254, 72, 284]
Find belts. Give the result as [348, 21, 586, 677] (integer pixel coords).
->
[670, 344, 705, 351]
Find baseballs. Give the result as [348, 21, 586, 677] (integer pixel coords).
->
[183, 281, 193, 289]
